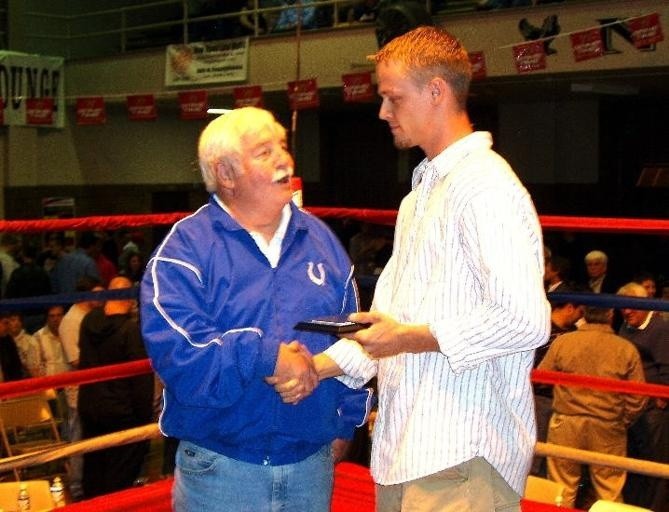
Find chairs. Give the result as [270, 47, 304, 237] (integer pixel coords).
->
[0, 388, 70, 482]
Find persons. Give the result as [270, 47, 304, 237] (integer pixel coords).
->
[225, 0, 539, 38]
[134, 104, 373, 512]
[348, 220, 392, 461]
[264, 26, 554, 512]
[533, 247, 669, 512]
[0, 230, 181, 501]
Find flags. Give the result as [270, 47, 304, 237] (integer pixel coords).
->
[342, 70, 376, 103]
[126, 93, 158, 122]
[75, 95, 107, 126]
[625, 14, 664, 50]
[232, 85, 265, 108]
[510, 40, 548, 73]
[178, 88, 210, 121]
[25, 95, 55, 123]
[468, 51, 489, 80]
[286, 78, 320, 110]
[569, 29, 605, 62]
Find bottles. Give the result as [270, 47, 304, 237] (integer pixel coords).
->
[18, 485, 31, 512]
[49, 476, 67, 509]
